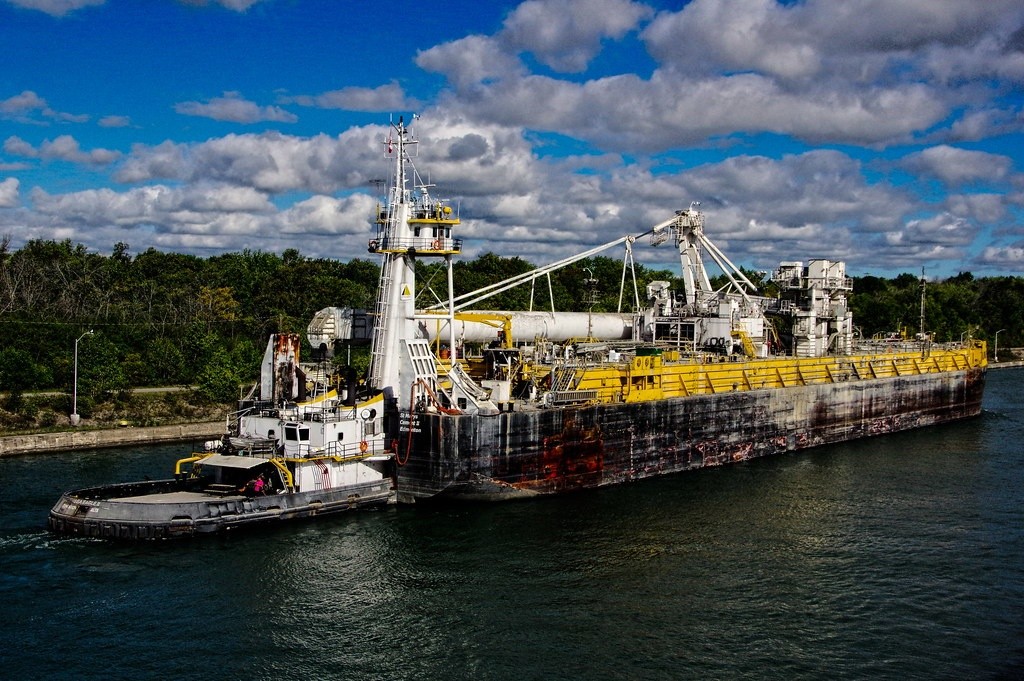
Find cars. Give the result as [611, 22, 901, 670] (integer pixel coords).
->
[884, 332, 902, 342]
[916, 331, 936, 341]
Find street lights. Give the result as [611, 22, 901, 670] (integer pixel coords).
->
[961, 330, 971, 345]
[71, 332, 94, 426]
[994, 328, 1006, 363]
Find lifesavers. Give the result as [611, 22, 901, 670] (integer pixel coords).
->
[359, 440, 368, 453]
[434, 240, 440, 250]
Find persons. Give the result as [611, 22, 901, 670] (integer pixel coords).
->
[252, 475, 264, 496]
